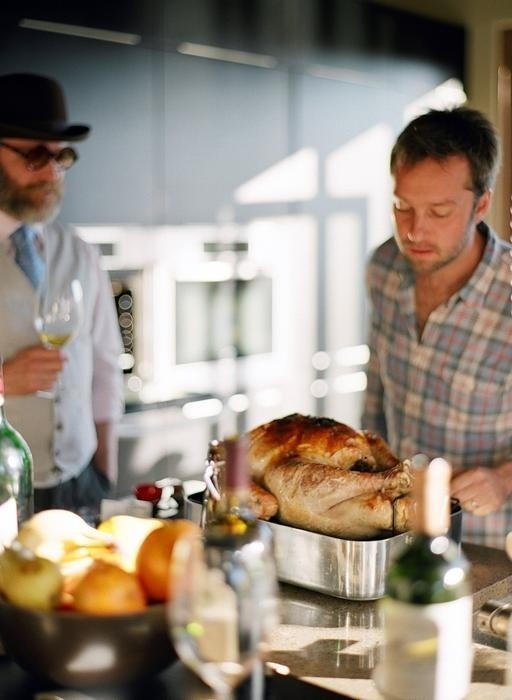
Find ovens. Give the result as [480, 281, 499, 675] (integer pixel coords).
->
[157, 220, 321, 402]
[59, 221, 157, 409]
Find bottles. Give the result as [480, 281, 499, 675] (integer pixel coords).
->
[0, 377, 35, 553]
[373, 452, 476, 699]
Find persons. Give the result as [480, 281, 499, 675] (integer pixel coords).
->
[1, 69, 133, 545]
[357, 103, 512, 555]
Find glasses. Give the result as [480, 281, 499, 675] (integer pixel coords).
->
[2, 142, 79, 171]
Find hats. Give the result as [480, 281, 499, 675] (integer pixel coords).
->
[0, 73, 90, 140]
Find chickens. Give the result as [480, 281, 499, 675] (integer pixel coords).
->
[206, 412, 419, 540]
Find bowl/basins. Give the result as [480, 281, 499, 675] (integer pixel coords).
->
[0, 588, 183, 699]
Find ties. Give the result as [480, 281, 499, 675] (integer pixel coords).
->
[14, 222, 45, 292]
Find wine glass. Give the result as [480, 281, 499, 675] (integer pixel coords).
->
[161, 512, 285, 699]
[28, 277, 83, 397]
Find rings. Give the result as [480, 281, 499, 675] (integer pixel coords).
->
[468, 499, 478, 510]
